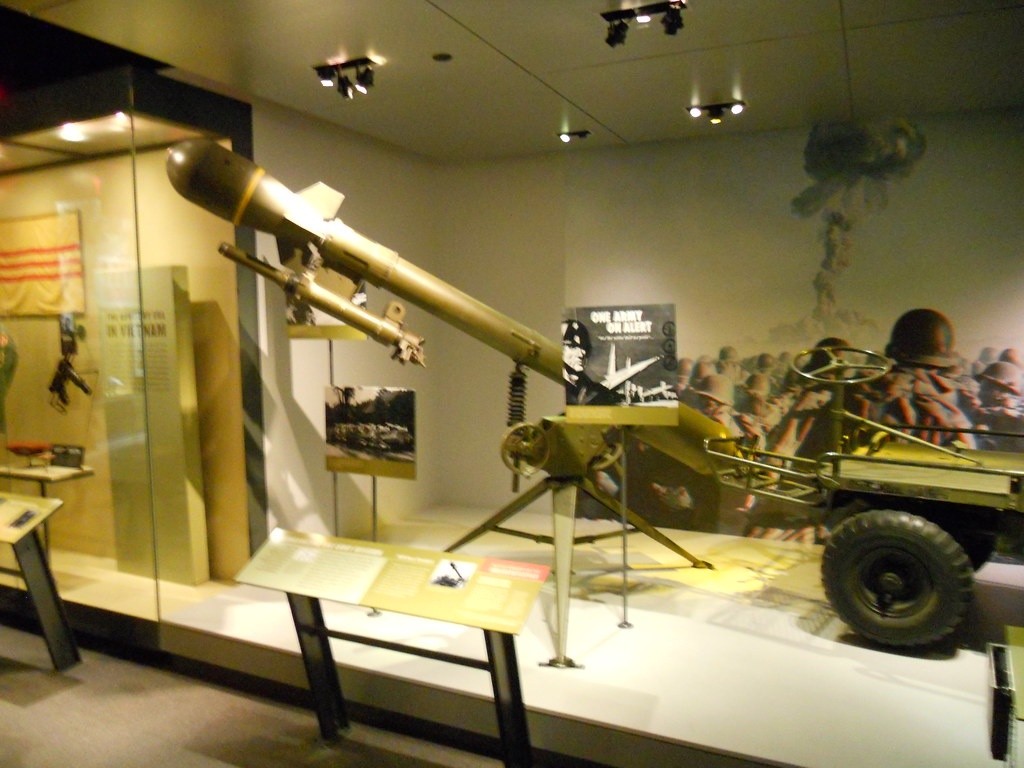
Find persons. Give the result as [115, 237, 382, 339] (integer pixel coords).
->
[675, 308, 1019, 546]
[561, 320, 625, 406]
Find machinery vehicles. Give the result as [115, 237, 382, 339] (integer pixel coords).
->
[165, 136, 1024, 654]
